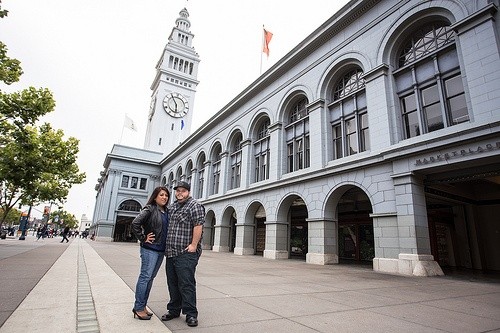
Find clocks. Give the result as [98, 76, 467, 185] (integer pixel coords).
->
[147, 95, 157, 121]
[162, 92, 190, 118]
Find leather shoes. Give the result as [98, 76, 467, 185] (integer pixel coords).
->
[186, 315, 198, 326]
[161, 311, 180, 320]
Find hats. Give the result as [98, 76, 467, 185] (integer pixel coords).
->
[173, 181, 190, 191]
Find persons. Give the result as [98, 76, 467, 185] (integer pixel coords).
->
[1, 225, 28, 236]
[34, 224, 96, 243]
[131, 186, 170, 320]
[161, 180, 206, 327]
[300, 244, 306, 255]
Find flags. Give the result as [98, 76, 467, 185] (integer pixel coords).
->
[263, 28, 273, 57]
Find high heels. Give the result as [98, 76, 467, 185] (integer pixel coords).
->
[147, 312, 153, 316]
[132, 307, 151, 320]
